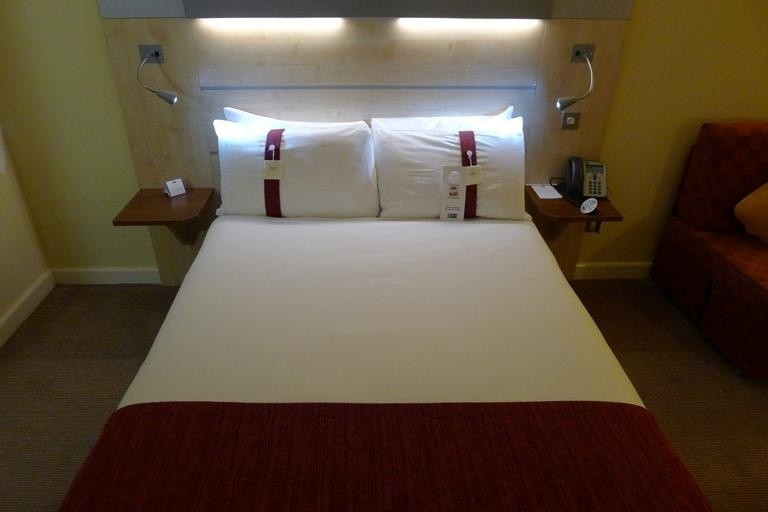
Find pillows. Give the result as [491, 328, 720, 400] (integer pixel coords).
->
[372, 105, 533, 220]
[212, 107, 371, 219]
[735, 183, 768, 245]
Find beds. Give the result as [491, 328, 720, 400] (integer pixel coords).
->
[55, 84, 715, 511]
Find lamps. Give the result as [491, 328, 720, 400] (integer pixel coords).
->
[137, 43, 178, 106]
[556, 44, 594, 111]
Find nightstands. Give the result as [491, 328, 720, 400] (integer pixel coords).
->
[112, 188, 215, 245]
[525, 183, 623, 233]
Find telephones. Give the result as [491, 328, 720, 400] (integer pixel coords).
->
[566, 157, 607, 201]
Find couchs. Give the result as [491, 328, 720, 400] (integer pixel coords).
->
[651, 119, 768, 382]
